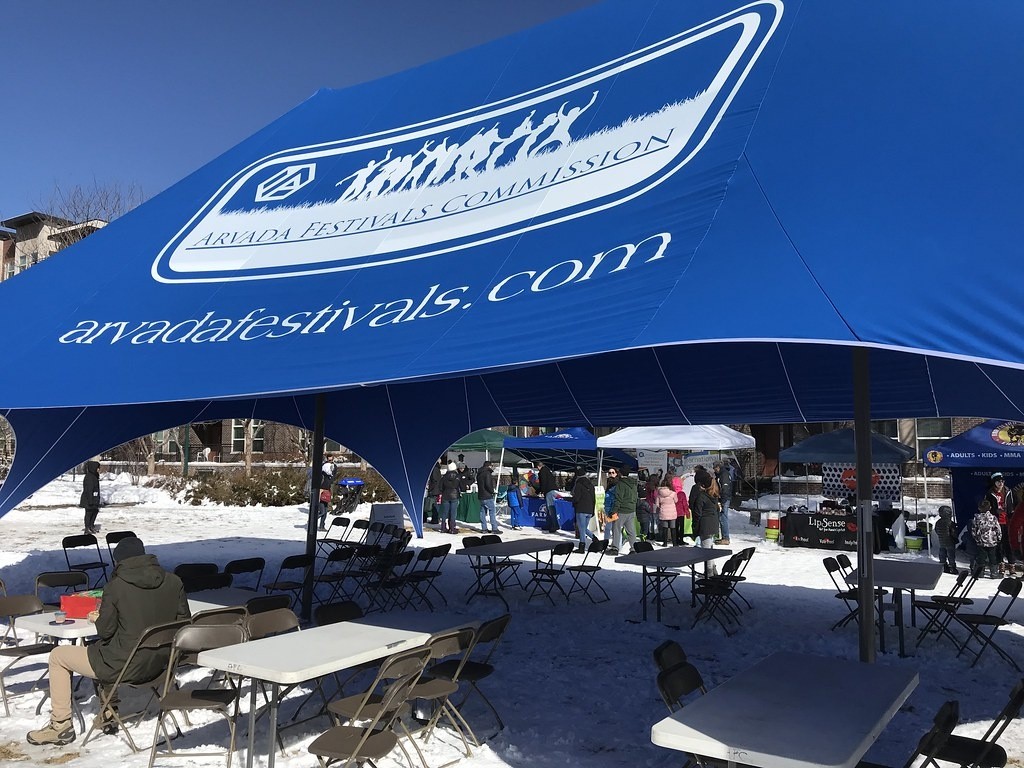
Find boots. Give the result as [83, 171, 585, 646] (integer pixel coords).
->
[662, 527, 668, 547]
[670, 528, 678, 547]
[441, 523, 449, 533]
[573, 542, 585, 553]
[448, 520, 459, 534]
[997, 562, 1017, 577]
[640, 534, 648, 542]
[592, 534, 599, 543]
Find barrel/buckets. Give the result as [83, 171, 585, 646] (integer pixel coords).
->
[767, 514, 778, 528]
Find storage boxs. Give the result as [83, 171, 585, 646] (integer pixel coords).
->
[59, 593, 102, 620]
[767, 513, 780, 529]
[903, 534, 925, 551]
[766, 538, 778, 544]
[764, 528, 779, 539]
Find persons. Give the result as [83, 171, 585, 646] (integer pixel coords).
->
[26, 537, 191, 746]
[935, 506, 960, 575]
[439, 460, 465, 534]
[604, 464, 691, 554]
[507, 477, 523, 530]
[570, 468, 599, 554]
[688, 461, 732, 579]
[423, 461, 441, 524]
[477, 461, 503, 534]
[322, 454, 337, 481]
[535, 460, 560, 533]
[317, 463, 333, 531]
[79, 461, 101, 535]
[456, 462, 475, 490]
[970, 472, 1024, 578]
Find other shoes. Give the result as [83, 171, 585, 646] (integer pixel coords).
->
[979, 571, 985, 577]
[89, 526, 97, 533]
[84, 530, 95, 535]
[715, 538, 729, 544]
[319, 527, 327, 531]
[679, 542, 690, 545]
[429, 519, 439, 524]
[991, 572, 1003, 579]
[606, 548, 618, 555]
[512, 525, 523, 530]
[492, 529, 503, 534]
[481, 529, 492, 533]
[944, 565, 959, 574]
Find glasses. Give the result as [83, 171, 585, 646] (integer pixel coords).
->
[608, 472, 616, 475]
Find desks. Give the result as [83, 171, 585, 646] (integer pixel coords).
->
[782, 509, 910, 555]
[195, 609, 490, 768]
[511, 496, 577, 533]
[844, 557, 946, 660]
[614, 545, 734, 623]
[649, 646, 922, 768]
[455, 538, 569, 612]
[10, 595, 231, 738]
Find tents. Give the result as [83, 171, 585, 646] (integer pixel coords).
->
[0, 0, 1024, 539]
[596, 424, 758, 498]
[922, 419, 1024, 558]
[778, 427, 917, 552]
[494, 427, 639, 504]
[446, 429, 506, 461]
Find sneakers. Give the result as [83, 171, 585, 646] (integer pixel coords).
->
[28, 718, 76, 746]
[93, 707, 120, 734]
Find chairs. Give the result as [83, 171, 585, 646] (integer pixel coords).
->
[0, 514, 1024, 768]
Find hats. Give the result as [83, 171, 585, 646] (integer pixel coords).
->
[578, 468, 586, 476]
[113, 537, 144, 564]
[447, 460, 457, 471]
[990, 472, 1005, 483]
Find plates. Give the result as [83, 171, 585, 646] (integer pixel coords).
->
[49, 620, 75, 625]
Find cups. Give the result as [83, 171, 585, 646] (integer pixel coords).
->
[55, 612, 66, 623]
